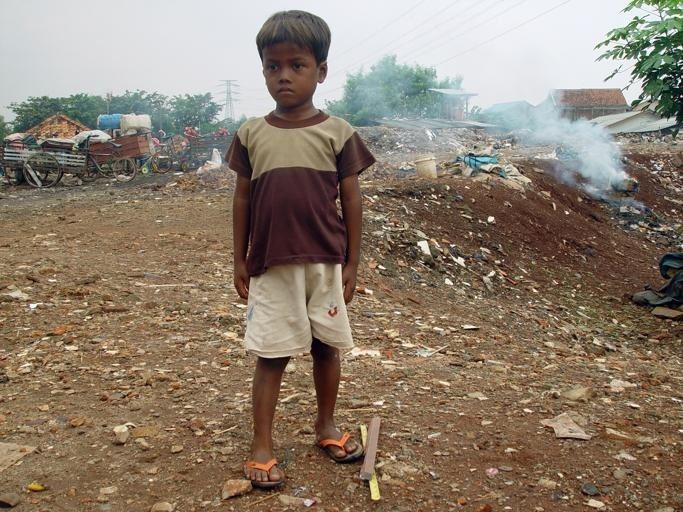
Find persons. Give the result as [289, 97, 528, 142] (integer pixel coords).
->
[224, 9, 377, 487]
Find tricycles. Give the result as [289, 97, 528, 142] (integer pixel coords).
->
[2, 131, 234, 189]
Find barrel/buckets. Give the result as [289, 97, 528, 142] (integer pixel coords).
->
[414, 154, 438, 179]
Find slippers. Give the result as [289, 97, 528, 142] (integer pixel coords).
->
[315, 429, 364, 463]
[245, 460, 283, 487]
